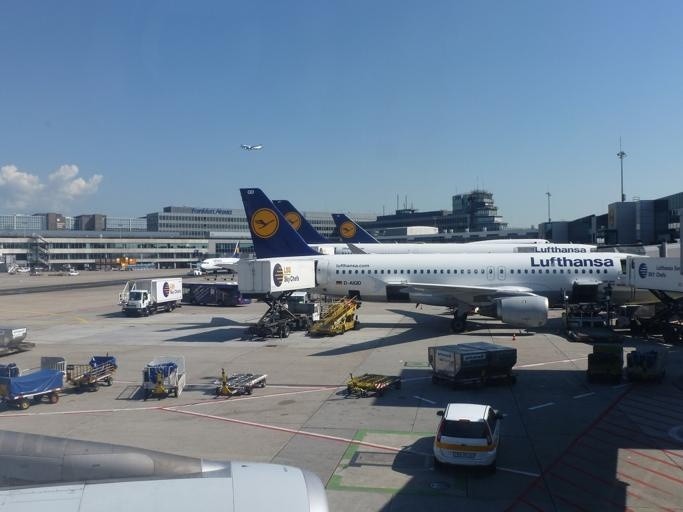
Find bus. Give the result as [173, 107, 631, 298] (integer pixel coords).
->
[181, 282, 251, 307]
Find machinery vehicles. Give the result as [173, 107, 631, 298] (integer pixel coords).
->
[311, 294, 360, 336]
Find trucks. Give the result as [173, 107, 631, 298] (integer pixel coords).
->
[123, 276, 183, 317]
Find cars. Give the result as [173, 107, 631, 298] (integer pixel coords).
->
[433, 401, 506, 467]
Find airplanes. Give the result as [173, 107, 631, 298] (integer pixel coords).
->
[240, 143, 263, 152]
[199, 239, 244, 273]
